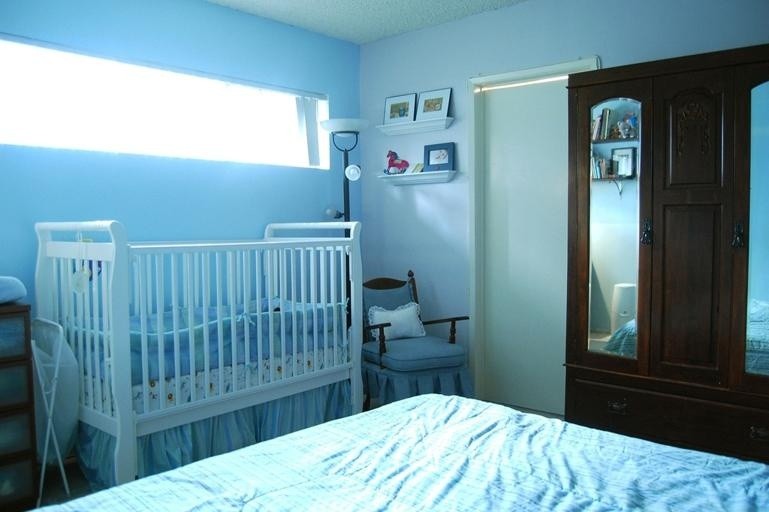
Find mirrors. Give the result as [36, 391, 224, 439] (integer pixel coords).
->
[576, 79, 652, 376]
[728, 70, 768, 394]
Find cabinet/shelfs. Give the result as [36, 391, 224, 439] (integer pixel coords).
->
[563, 43, 769, 466]
[0, 302, 38, 512]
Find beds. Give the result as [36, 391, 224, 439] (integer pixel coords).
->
[35, 220, 362, 486]
[25, 392, 769, 512]
[604, 298, 768, 373]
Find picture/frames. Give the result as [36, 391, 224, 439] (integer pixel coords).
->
[611, 147, 636, 178]
[424, 142, 455, 171]
[383, 93, 416, 125]
[415, 87, 452, 121]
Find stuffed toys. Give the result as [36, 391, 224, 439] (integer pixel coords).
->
[383, 150, 409, 175]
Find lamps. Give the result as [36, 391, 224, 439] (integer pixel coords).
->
[322, 118, 369, 328]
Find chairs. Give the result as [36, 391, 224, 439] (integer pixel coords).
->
[361, 270, 469, 411]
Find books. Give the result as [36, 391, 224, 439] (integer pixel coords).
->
[592, 155, 606, 178]
[592, 108, 610, 140]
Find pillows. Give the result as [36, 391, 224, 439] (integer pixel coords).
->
[369, 302, 426, 339]
[0, 277, 26, 302]
